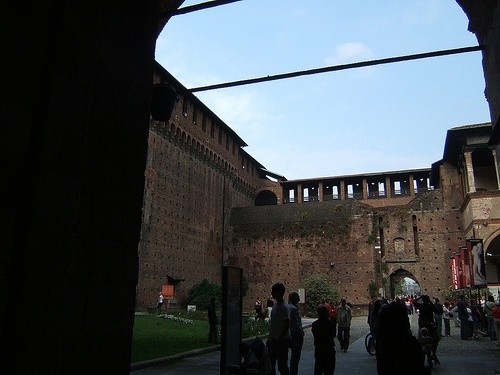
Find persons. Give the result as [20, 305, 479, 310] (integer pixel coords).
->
[412, 295, 441, 365]
[465, 303, 476, 339]
[376, 302, 431, 375]
[312, 307, 336, 375]
[420, 328, 436, 369]
[288, 291, 305, 374]
[442, 300, 451, 337]
[492, 303, 500, 340]
[254, 293, 354, 338]
[265, 283, 291, 375]
[470, 300, 483, 339]
[458, 302, 470, 340]
[239, 343, 264, 375]
[157, 291, 164, 310]
[369, 294, 483, 330]
[472, 242, 487, 286]
[483, 296, 496, 337]
[368, 301, 381, 351]
[336, 297, 353, 352]
[207, 295, 219, 344]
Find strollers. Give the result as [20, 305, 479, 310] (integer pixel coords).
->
[226, 336, 276, 375]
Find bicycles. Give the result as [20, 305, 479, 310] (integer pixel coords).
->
[365, 332, 377, 355]
[418, 317, 437, 369]
